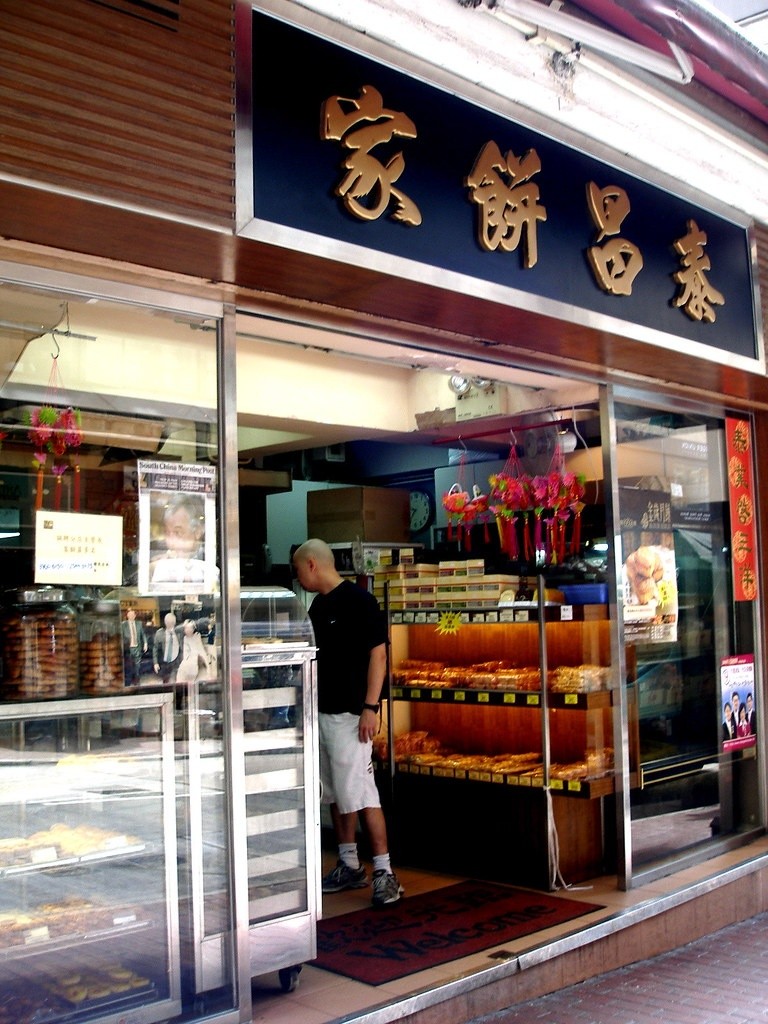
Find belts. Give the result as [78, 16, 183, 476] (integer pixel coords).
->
[163, 656, 179, 664]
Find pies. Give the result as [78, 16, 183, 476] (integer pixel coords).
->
[46, 963, 147, 1000]
[2, 612, 123, 697]
[627, 547, 664, 602]
[0, 896, 144, 948]
[0, 823, 141, 864]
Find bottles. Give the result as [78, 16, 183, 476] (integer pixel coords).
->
[514, 575, 533, 601]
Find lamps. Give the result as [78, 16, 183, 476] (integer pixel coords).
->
[448, 374, 508, 422]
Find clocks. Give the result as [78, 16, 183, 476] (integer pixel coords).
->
[410, 491, 435, 532]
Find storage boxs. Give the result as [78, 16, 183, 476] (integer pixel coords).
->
[307, 486, 411, 543]
[372, 547, 538, 608]
[558, 583, 608, 604]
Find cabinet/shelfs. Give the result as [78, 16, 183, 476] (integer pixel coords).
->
[0, 692, 186, 1024]
[71, 644, 325, 997]
[386, 604, 616, 887]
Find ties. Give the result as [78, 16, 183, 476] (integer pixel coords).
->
[167, 632, 173, 663]
[132, 623, 136, 645]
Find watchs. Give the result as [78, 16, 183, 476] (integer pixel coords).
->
[364, 703, 380, 714]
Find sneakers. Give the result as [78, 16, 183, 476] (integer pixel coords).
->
[371, 869, 405, 905]
[322, 860, 368, 892]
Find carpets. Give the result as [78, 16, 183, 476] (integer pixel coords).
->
[302, 880, 607, 989]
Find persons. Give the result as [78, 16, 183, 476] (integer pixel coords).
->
[149, 491, 205, 581]
[123, 608, 216, 685]
[722, 691, 757, 742]
[293, 539, 405, 907]
